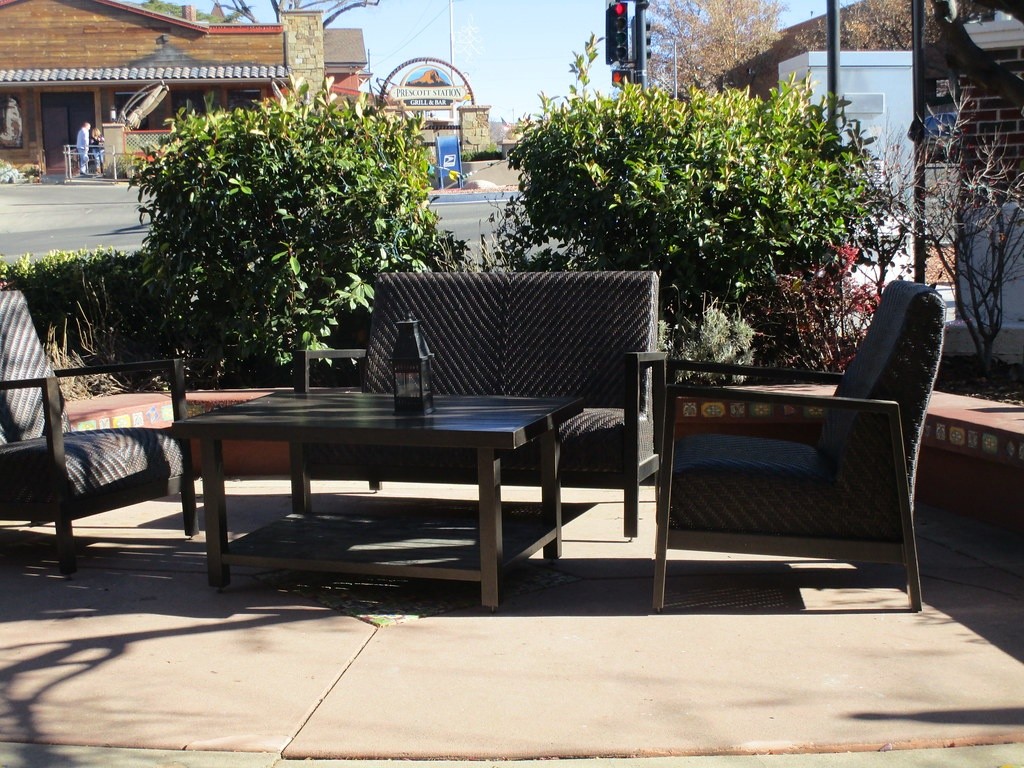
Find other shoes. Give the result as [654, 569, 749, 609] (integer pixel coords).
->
[94, 168, 101, 174]
[80, 172, 88, 176]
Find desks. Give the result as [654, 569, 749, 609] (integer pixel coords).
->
[171, 385, 584, 615]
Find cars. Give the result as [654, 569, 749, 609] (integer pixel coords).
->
[925, 113, 961, 163]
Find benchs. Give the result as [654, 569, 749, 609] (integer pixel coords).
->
[291, 272, 656, 542]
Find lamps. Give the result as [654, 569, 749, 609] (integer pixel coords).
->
[110, 105, 116, 122]
[388, 310, 434, 417]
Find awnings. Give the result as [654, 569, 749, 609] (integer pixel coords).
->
[0, 66, 294, 85]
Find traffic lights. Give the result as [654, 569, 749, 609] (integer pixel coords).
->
[610, 1, 628, 62]
[646, 18, 652, 60]
[612, 71, 631, 88]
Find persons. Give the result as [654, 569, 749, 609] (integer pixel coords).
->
[90, 128, 105, 174]
[77, 122, 91, 175]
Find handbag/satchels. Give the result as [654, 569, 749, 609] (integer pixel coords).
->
[74, 147, 79, 161]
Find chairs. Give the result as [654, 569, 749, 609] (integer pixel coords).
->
[0, 291, 199, 581]
[652, 280, 947, 611]
